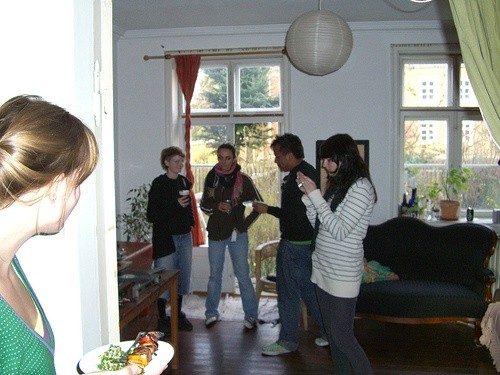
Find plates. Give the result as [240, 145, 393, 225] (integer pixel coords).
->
[78, 340, 174, 375]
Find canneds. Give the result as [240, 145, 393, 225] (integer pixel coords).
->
[467, 207, 474, 221]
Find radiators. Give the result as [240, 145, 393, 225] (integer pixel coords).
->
[489, 238, 500, 299]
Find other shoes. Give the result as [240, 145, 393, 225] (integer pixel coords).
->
[158, 316, 171, 337]
[205, 317, 217, 327]
[244, 321, 253, 329]
[178, 312, 194, 331]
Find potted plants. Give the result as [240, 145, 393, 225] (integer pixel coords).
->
[407, 168, 472, 219]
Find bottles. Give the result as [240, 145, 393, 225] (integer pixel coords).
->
[432, 209, 440, 222]
[401, 193, 409, 216]
[408, 187, 418, 218]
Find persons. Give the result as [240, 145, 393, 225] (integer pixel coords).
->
[200, 144, 265, 329]
[0, 95, 170, 374]
[147, 146, 196, 338]
[295, 133, 377, 375]
[252, 132, 328, 356]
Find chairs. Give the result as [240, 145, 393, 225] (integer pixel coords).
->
[255, 240, 308, 331]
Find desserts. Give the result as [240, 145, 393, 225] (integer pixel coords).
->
[98, 344, 126, 370]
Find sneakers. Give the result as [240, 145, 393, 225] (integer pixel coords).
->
[315, 338, 329, 347]
[261, 342, 291, 355]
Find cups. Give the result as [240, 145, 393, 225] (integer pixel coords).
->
[179, 190, 189, 201]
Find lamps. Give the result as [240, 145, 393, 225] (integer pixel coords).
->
[286, 0, 353, 76]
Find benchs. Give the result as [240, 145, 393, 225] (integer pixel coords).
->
[355, 216, 497, 348]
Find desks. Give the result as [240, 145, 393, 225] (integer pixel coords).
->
[118, 269, 180, 369]
